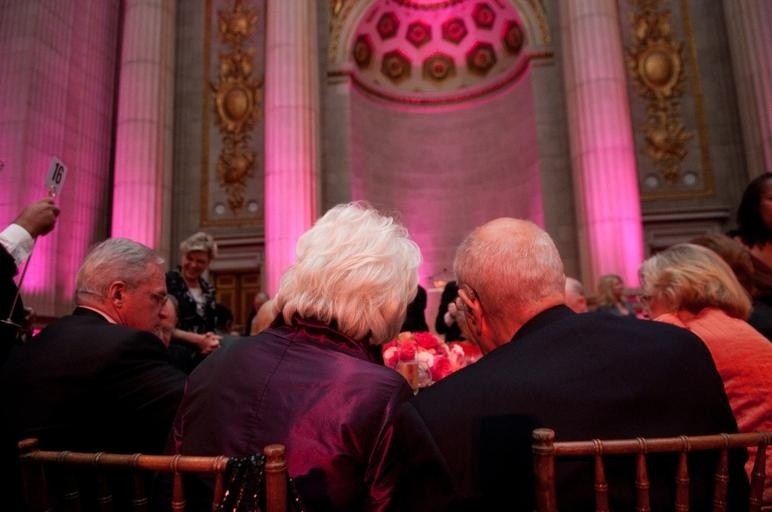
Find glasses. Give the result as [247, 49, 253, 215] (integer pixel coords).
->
[636, 292, 659, 311]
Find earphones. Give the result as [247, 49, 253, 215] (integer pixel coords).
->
[114, 290, 121, 299]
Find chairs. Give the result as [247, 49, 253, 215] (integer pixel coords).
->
[16, 437, 288, 511]
[531, 428, 772, 512]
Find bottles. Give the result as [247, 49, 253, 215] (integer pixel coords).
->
[396, 320, 419, 397]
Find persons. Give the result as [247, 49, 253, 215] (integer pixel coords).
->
[414, 217, 772, 512]
[726, 173, 772, 339]
[1, 197, 453, 512]
